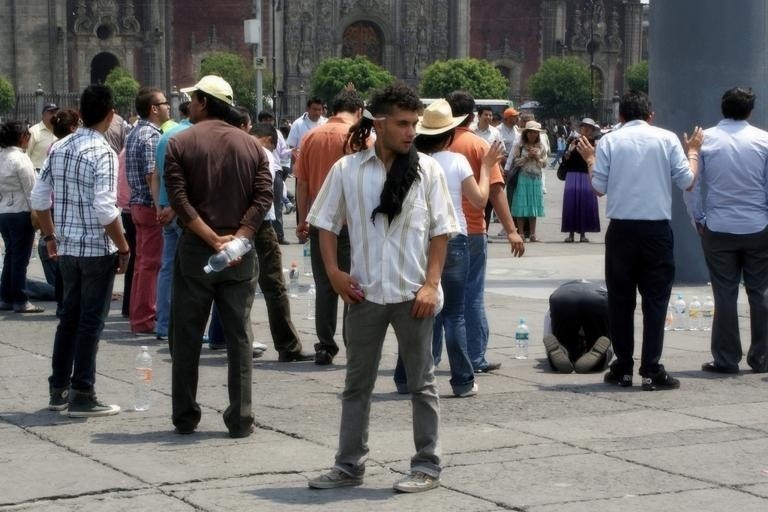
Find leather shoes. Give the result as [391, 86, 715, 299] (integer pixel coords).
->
[277, 350, 316, 363]
[701, 360, 740, 374]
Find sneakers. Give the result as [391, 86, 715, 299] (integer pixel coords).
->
[394, 376, 479, 397]
[574, 334, 612, 373]
[65, 390, 122, 419]
[48, 382, 72, 412]
[540, 332, 575, 375]
[390, 469, 442, 494]
[474, 361, 502, 373]
[640, 362, 681, 390]
[306, 466, 365, 491]
[601, 367, 635, 387]
[282, 199, 298, 216]
[314, 348, 334, 365]
[13, 303, 45, 312]
[101, 291, 274, 360]
[497, 227, 595, 246]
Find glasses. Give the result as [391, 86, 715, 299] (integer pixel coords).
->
[155, 100, 169, 107]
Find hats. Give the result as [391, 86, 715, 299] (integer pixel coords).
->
[573, 117, 602, 139]
[41, 102, 59, 116]
[501, 106, 521, 119]
[413, 97, 471, 139]
[513, 121, 546, 134]
[178, 73, 236, 106]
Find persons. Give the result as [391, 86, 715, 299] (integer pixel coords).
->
[304, 82, 461, 495]
[28, 85, 133, 419]
[682, 86, 768, 375]
[162, 74, 273, 441]
[574, 88, 706, 390]
[0, 81, 620, 400]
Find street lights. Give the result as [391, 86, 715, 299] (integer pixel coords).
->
[588, 12, 605, 120]
[271, 0, 285, 129]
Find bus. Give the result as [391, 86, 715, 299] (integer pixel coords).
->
[356, 96, 519, 132]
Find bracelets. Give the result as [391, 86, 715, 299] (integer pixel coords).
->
[687, 152, 698, 156]
[688, 157, 698, 162]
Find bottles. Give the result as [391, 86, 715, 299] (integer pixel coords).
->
[304, 240, 313, 277]
[289, 261, 299, 298]
[666, 294, 716, 330]
[133, 345, 151, 410]
[204, 235, 252, 274]
[307, 284, 316, 319]
[516, 320, 529, 359]
